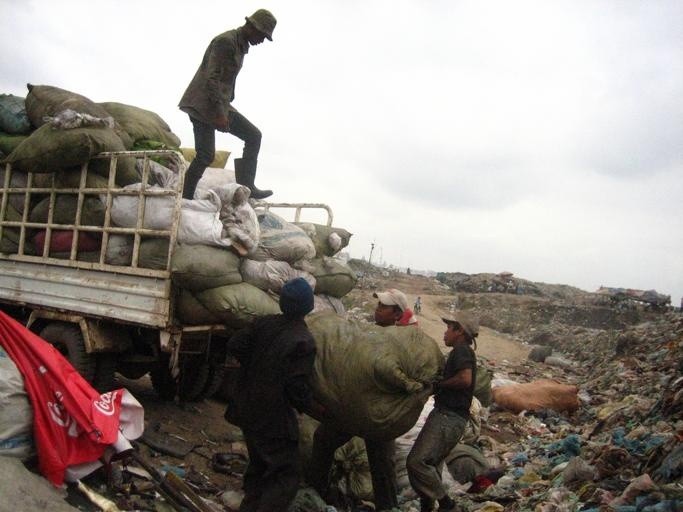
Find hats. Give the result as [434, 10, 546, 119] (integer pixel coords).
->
[373, 289, 408, 313]
[442, 312, 479, 337]
[279, 278, 314, 315]
[245, 10, 276, 41]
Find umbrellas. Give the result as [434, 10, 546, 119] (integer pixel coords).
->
[0, 309, 201, 512]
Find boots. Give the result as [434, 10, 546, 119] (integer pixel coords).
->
[235, 158, 273, 199]
[182, 158, 209, 200]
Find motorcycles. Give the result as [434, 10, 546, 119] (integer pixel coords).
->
[414, 302, 422, 313]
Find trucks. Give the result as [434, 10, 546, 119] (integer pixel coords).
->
[0, 149, 333, 404]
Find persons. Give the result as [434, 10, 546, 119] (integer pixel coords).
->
[413, 297, 421, 312]
[406, 312, 479, 512]
[223, 277, 340, 512]
[303, 288, 408, 512]
[178, 8, 273, 199]
